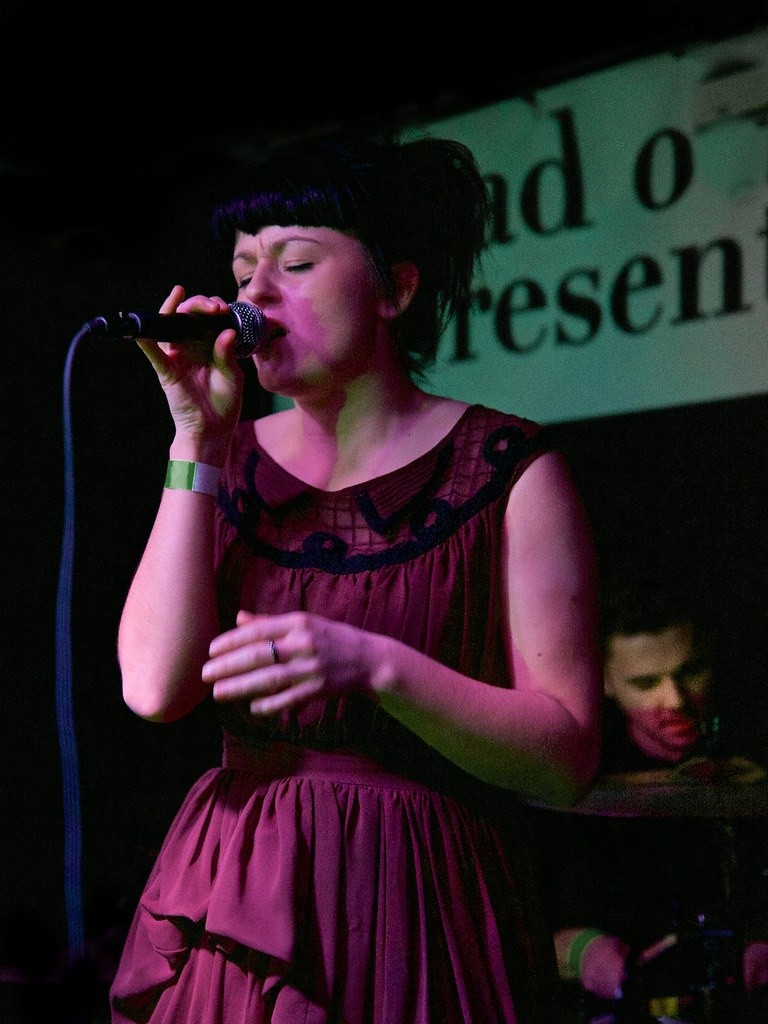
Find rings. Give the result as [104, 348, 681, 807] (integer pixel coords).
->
[269, 639, 280, 665]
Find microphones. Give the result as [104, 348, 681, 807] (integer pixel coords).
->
[84, 301, 269, 358]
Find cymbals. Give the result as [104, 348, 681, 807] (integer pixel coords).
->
[513, 767, 768, 821]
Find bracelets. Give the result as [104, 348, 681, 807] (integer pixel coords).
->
[166, 459, 224, 498]
[567, 929, 599, 980]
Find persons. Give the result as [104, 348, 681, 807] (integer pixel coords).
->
[553, 586, 768, 1024]
[110, 139, 606, 1024]
